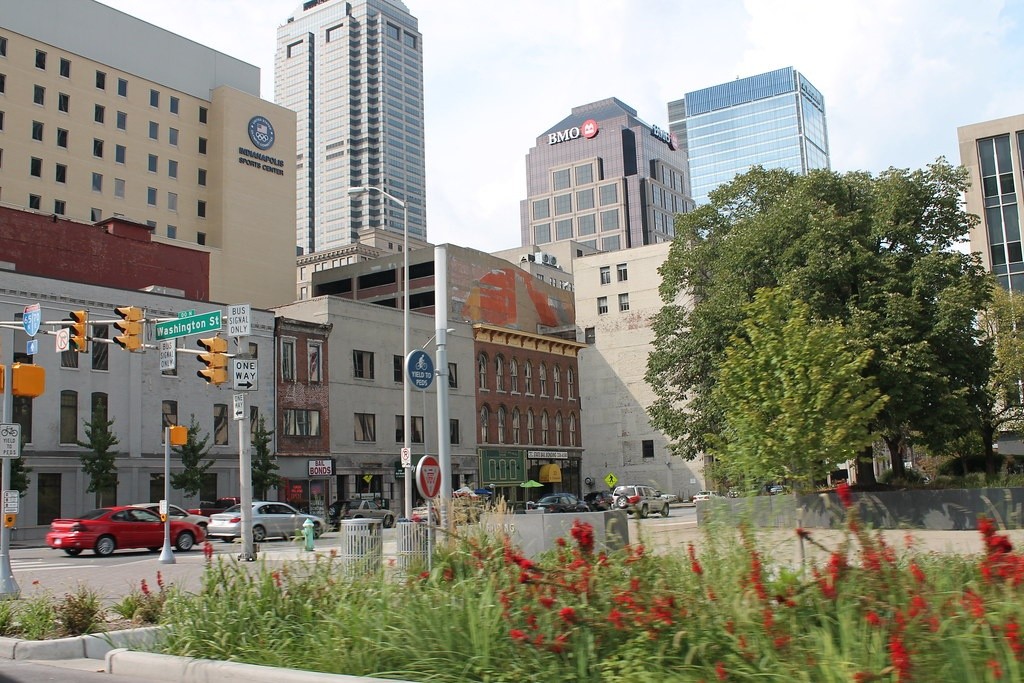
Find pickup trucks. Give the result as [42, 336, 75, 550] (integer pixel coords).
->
[413, 498, 482, 525]
[187, 497, 240, 517]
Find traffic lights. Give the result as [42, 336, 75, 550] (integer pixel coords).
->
[68, 310, 88, 355]
[196, 338, 229, 387]
[111, 305, 143, 354]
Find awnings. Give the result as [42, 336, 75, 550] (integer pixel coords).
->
[531, 464, 562, 482]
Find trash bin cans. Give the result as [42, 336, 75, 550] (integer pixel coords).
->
[395, 521, 437, 566]
[339, 519, 384, 578]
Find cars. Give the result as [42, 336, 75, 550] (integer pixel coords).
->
[727, 484, 787, 499]
[126, 503, 210, 539]
[532, 484, 679, 518]
[44, 506, 206, 557]
[206, 501, 325, 542]
[692, 490, 726, 504]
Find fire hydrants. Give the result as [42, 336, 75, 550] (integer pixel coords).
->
[301, 518, 315, 551]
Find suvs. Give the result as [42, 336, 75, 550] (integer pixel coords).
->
[611, 485, 669, 518]
[328, 499, 396, 532]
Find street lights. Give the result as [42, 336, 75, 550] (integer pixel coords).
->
[346, 185, 411, 523]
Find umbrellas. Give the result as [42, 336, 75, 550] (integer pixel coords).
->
[453, 487, 493, 497]
[521, 480, 544, 499]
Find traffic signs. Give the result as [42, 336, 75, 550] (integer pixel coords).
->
[232, 394, 245, 420]
[232, 360, 258, 391]
